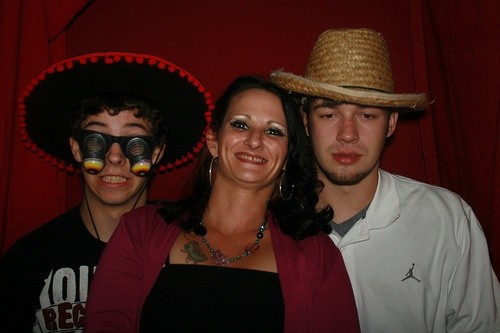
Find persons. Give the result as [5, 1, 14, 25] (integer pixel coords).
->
[1, 50, 214, 332]
[82, 73, 362, 333]
[270, 27, 500, 333]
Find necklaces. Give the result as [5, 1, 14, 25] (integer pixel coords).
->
[198, 220, 268, 262]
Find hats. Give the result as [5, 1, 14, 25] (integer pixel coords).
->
[17, 52, 213, 173]
[271, 28, 428, 110]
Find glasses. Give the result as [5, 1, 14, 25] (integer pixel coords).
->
[73, 129, 159, 176]
